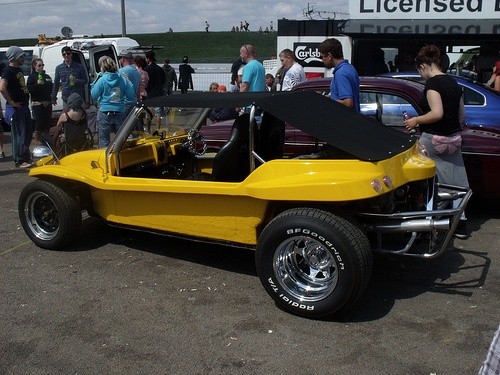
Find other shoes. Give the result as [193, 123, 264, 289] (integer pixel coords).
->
[18, 162, 32, 168]
[0, 152, 5, 159]
[453, 224, 469, 238]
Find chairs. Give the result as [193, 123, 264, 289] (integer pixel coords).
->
[53, 122, 89, 159]
[211, 111, 258, 182]
[256, 111, 286, 166]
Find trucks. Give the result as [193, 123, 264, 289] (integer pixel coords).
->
[0, 36, 166, 130]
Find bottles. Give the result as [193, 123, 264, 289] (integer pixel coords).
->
[69, 74, 74, 86]
[38, 73, 43, 85]
[402, 110, 417, 135]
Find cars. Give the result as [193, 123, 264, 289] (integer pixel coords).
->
[19, 92, 473, 322]
[176, 75, 500, 208]
[359, 72, 500, 131]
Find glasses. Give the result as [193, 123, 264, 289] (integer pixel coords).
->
[38, 64, 44, 67]
[63, 52, 71, 56]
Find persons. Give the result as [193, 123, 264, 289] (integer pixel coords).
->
[403, 44, 470, 239]
[0, 45, 31, 167]
[209, 85, 235, 123]
[275, 65, 287, 91]
[27, 47, 177, 151]
[205, 20, 276, 34]
[317, 38, 360, 113]
[207, 81, 218, 117]
[279, 49, 306, 91]
[231, 58, 244, 90]
[489, 60, 500, 91]
[266, 74, 276, 91]
[235, 44, 266, 116]
[178, 56, 195, 94]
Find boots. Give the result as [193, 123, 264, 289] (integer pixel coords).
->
[34, 131, 43, 145]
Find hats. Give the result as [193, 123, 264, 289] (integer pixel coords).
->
[145, 49, 157, 62]
[6, 46, 26, 62]
[67, 92, 82, 109]
[116, 49, 134, 59]
